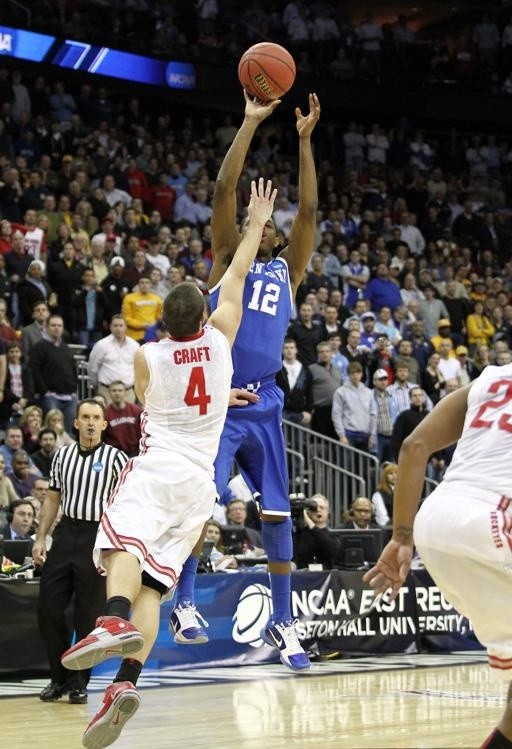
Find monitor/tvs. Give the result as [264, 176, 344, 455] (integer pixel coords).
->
[328, 528, 383, 568]
[0, 539, 35, 574]
[222, 526, 246, 547]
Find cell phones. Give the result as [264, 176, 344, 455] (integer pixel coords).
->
[378, 338, 383, 343]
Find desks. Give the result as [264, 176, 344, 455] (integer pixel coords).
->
[0, 568, 486, 679]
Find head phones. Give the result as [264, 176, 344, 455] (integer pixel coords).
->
[348, 495, 376, 520]
[6, 499, 36, 523]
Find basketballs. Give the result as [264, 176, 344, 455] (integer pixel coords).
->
[238, 43, 297, 100]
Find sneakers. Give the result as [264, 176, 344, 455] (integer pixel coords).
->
[40, 682, 70, 700]
[60, 616, 145, 671]
[82, 680, 141, 749]
[260, 615, 311, 672]
[69, 687, 88, 704]
[168, 598, 209, 644]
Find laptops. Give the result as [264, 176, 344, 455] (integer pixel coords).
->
[199, 540, 215, 564]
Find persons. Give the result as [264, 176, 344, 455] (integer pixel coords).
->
[362, 365, 510, 748]
[168, 94, 320, 672]
[60, 178, 278, 746]
[1, 1, 511, 570]
[32, 399, 131, 703]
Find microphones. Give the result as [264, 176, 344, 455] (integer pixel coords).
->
[30, 520, 38, 530]
[364, 517, 375, 521]
[317, 512, 331, 520]
